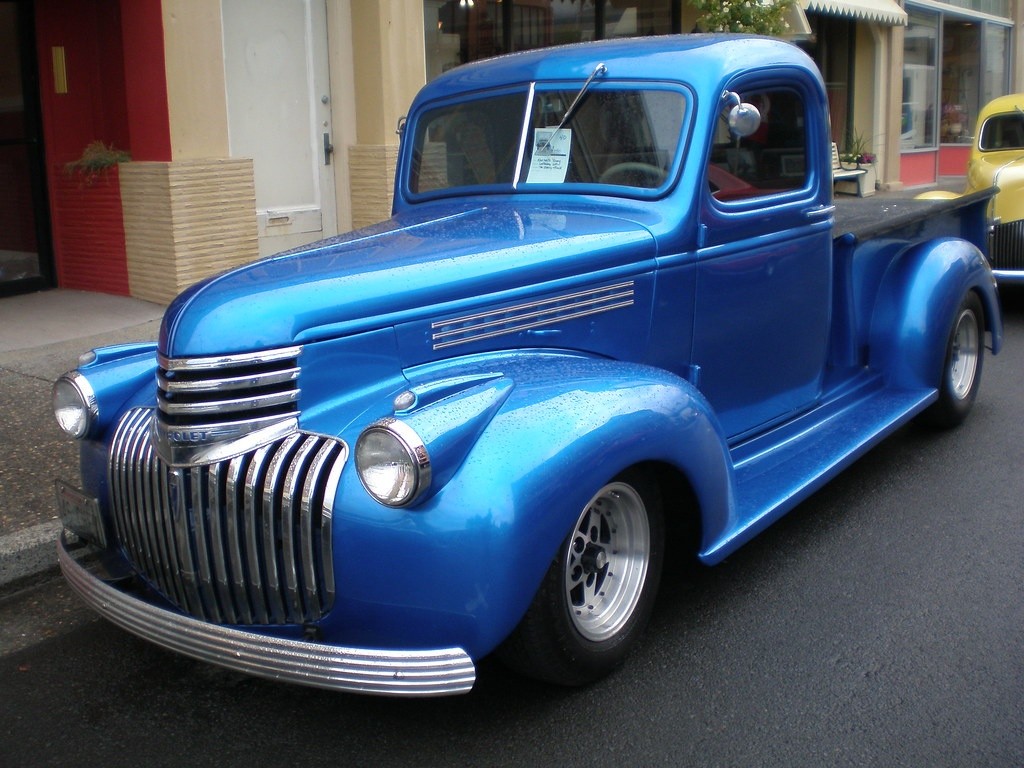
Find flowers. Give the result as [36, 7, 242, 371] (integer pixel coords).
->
[943, 103, 964, 122]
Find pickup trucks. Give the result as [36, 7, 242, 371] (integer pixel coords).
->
[49, 30, 1005, 702]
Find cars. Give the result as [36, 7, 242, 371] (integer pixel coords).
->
[911, 92, 1024, 296]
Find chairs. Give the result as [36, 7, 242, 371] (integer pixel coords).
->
[832, 142, 868, 199]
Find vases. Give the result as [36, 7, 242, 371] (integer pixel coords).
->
[949, 123, 962, 134]
